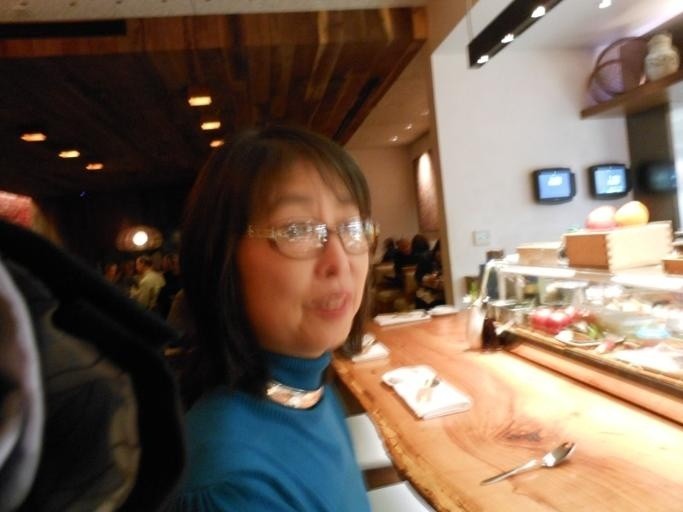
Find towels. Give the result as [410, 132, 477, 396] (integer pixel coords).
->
[382, 364, 472, 418]
[374, 308, 432, 326]
[352, 342, 389, 363]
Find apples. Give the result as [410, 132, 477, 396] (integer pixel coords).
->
[529, 304, 576, 334]
[586, 205, 619, 230]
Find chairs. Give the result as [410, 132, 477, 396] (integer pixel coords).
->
[394, 267, 416, 312]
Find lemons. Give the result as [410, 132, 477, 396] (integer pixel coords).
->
[613, 200, 649, 225]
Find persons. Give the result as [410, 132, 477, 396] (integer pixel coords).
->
[379, 233, 444, 310]
[107, 253, 182, 307]
[169, 130, 378, 511]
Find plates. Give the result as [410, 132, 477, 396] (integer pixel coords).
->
[555, 322, 619, 350]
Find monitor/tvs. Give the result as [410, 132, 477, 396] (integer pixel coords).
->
[533, 168, 576, 202]
[638, 160, 677, 194]
[589, 164, 632, 199]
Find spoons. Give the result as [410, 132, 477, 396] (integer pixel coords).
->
[479, 442, 577, 487]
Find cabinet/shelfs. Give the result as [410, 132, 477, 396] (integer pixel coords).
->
[479, 258, 683, 424]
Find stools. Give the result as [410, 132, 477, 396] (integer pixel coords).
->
[376, 288, 399, 313]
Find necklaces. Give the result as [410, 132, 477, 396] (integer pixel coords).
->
[265, 379, 324, 411]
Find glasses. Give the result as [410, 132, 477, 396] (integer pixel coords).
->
[246, 217, 380, 260]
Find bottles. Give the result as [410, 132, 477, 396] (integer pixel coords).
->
[465, 303, 483, 350]
[644, 35, 677, 84]
